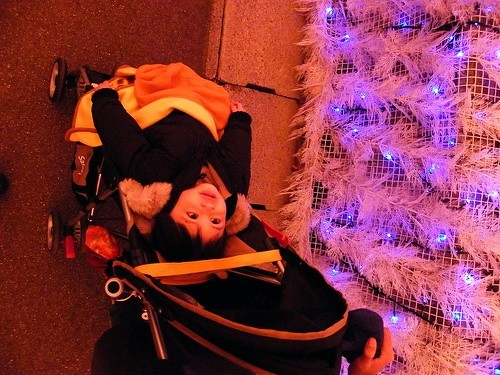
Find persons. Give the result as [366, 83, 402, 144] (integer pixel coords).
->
[348, 327, 394, 375]
[65, 64, 253, 263]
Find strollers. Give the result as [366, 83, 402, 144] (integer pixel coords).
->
[48, 57, 383, 374]
